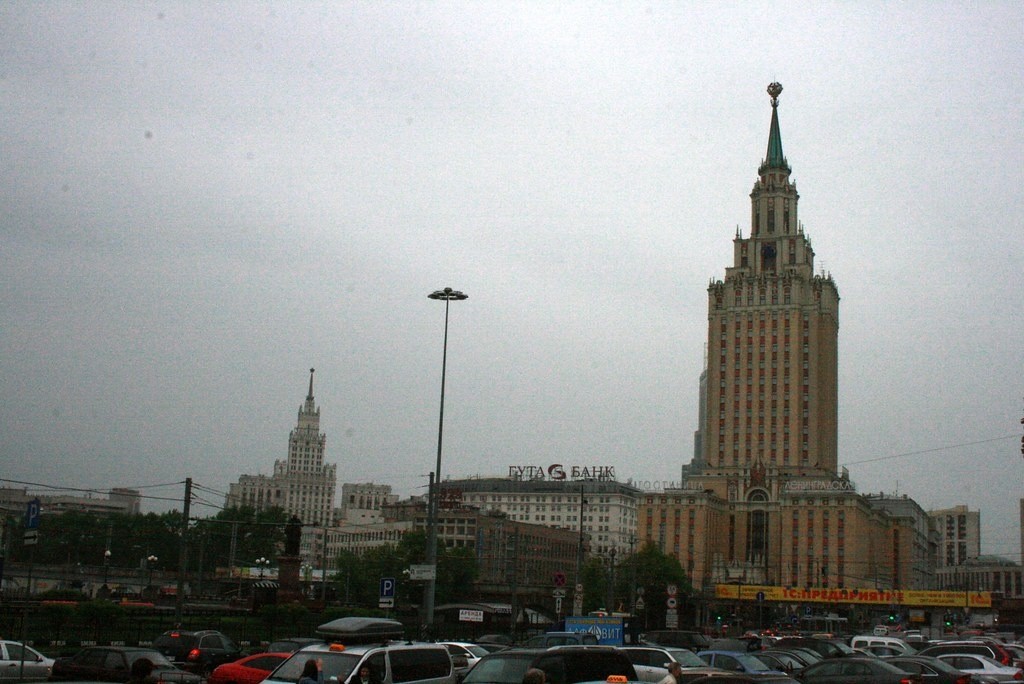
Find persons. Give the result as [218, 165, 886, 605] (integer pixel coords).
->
[522, 668, 546, 684]
[657, 662, 682, 684]
[747, 636, 762, 653]
[125, 657, 154, 684]
[296, 657, 324, 684]
[357, 662, 379, 684]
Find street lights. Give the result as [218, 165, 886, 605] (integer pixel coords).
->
[889, 610, 895, 626]
[148, 555, 158, 602]
[421, 287, 469, 639]
[102, 551, 112, 585]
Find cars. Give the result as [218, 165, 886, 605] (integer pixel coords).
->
[51, 646, 202, 684]
[264, 638, 325, 655]
[695, 650, 788, 677]
[788, 655, 922, 684]
[433, 642, 508, 683]
[881, 655, 972, 684]
[616, 645, 742, 683]
[207, 652, 342, 684]
[0, 641, 56, 684]
[936, 653, 1024, 684]
[943, 617, 1024, 668]
[743, 614, 937, 659]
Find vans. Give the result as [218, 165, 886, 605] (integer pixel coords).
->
[258, 617, 455, 684]
[645, 631, 711, 655]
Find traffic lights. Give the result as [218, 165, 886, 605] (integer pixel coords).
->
[946, 615, 951, 630]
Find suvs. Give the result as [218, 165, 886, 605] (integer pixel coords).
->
[913, 639, 1014, 667]
[149, 629, 250, 679]
[496, 631, 599, 657]
[460, 645, 639, 684]
